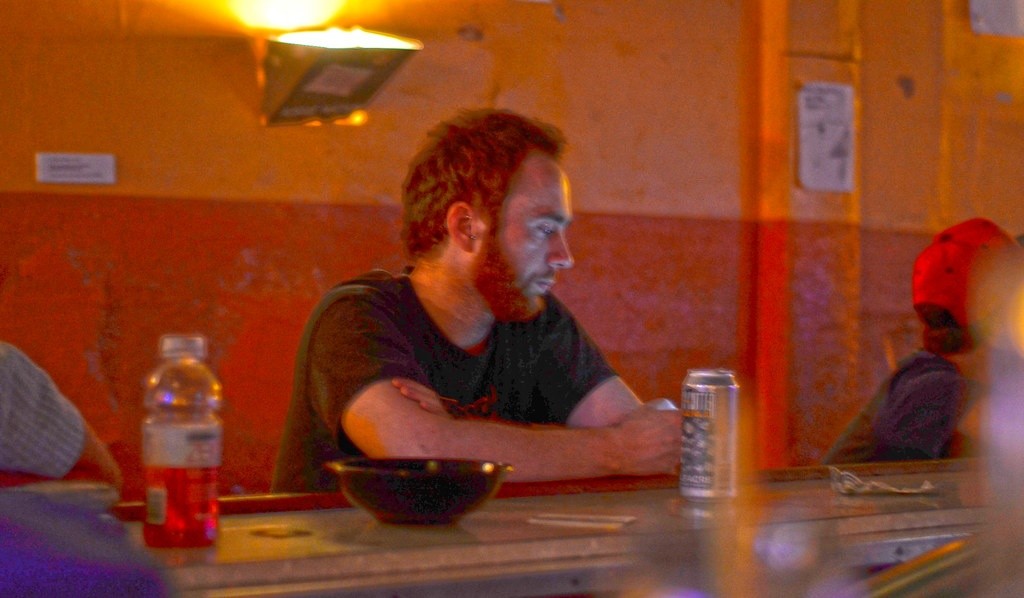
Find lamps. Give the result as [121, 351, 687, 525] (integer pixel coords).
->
[262, 23, 425, 126]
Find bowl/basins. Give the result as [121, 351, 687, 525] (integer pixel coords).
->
[322, 456, 517, 529]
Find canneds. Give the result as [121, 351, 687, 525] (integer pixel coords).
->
[680, 367, 740, 502]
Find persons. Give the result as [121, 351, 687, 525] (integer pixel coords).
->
[824, 217, 1024, 464]
[0, 339, 123, 504]
[275, 109, 684, 495]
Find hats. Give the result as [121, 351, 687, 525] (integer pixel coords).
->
[912, 217, 1024, 337]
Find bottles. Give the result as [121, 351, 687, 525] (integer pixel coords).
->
[143, 334, 223, 547]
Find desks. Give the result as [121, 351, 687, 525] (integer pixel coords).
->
[121, 452, 986, 598]
